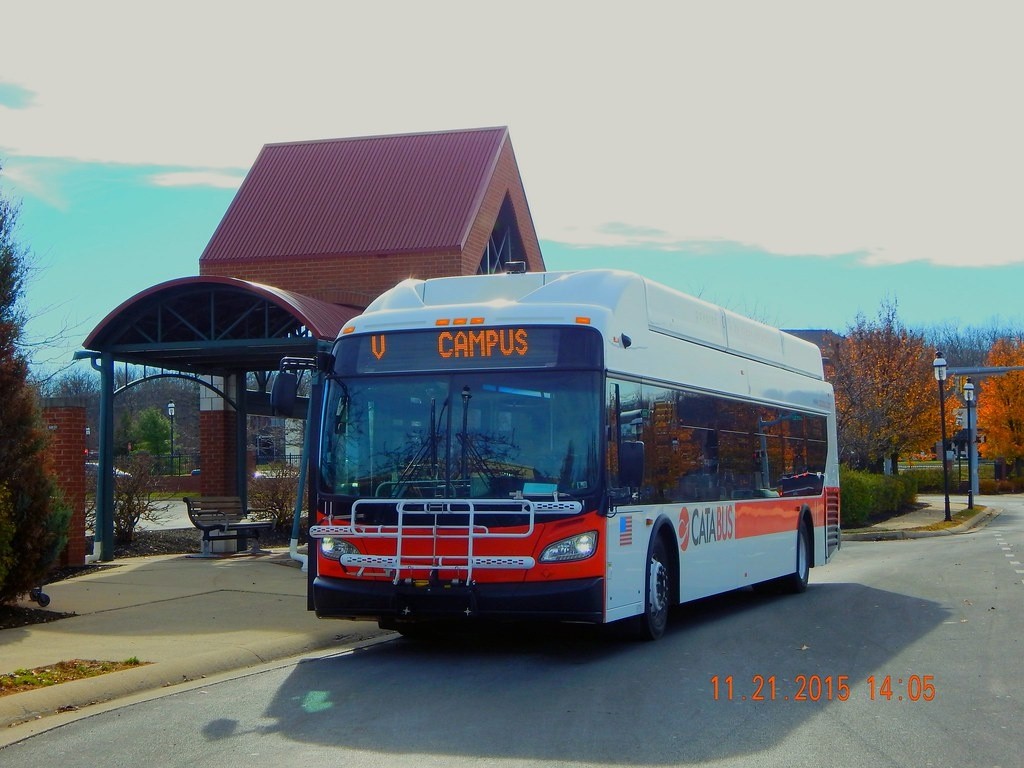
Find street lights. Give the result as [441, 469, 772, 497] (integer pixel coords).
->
[963, 376, 974, 509]
[933, 351, 953, 522]
[167, 400, 175, 475]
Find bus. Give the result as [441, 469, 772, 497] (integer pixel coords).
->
[269, 263, 842, 650]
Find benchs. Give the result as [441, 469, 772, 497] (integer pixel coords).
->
[182, 497, 273, 558]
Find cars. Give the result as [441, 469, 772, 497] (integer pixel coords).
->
[84, 462, 132, 480]
[85, 451, 100, 461]
